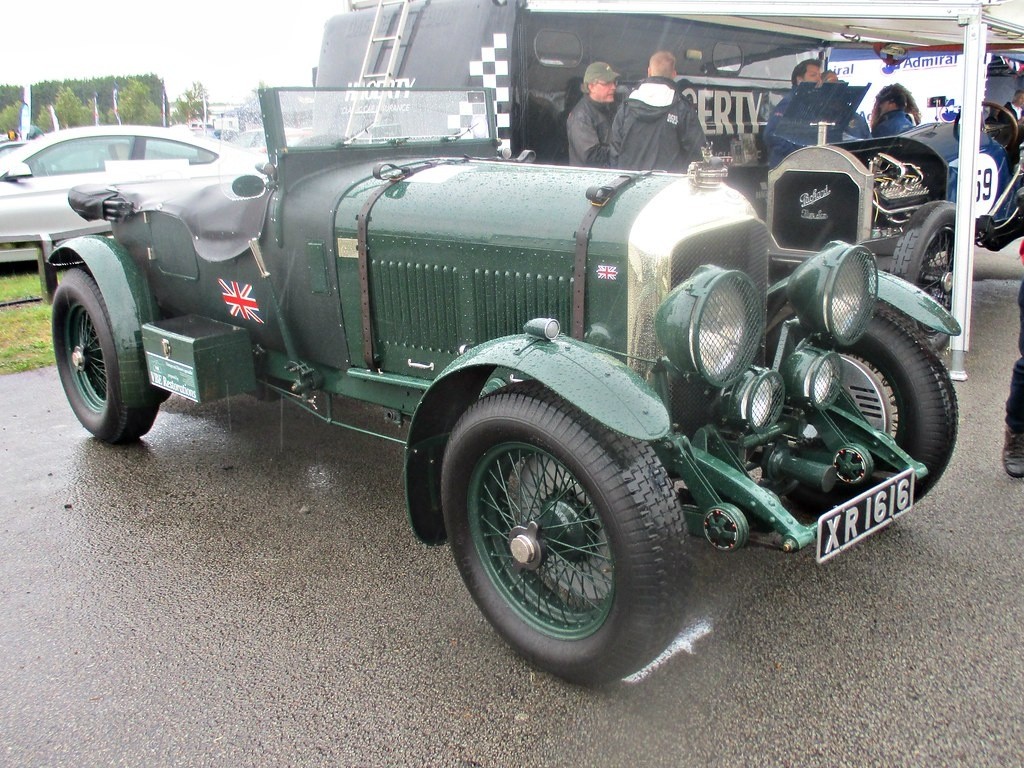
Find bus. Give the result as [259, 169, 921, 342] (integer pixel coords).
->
[301, 2, 927, 168]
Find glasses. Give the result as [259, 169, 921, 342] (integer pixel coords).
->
[596, 79, 620, 87]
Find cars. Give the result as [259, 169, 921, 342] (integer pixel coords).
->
[47, 83, 964, 687]
[0, 122, 309, 266]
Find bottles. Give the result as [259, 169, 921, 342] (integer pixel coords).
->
[730, 134, 758, 163]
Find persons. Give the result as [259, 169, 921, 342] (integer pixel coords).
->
[869, 82, 921, 142]
[1003, 235, 1024, 479]
[566, 60, 626, 169]
[611, 51, 712, 174]
[869, 86, 915, 138]
[983, 89, 1024, 177]
[820, 70, 872, 144]
[762, 58, 848, 168]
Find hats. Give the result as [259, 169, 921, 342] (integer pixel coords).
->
[583, 62, 621, 82]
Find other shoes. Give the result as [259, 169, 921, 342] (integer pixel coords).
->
[1002, 425, 1024, 478]
